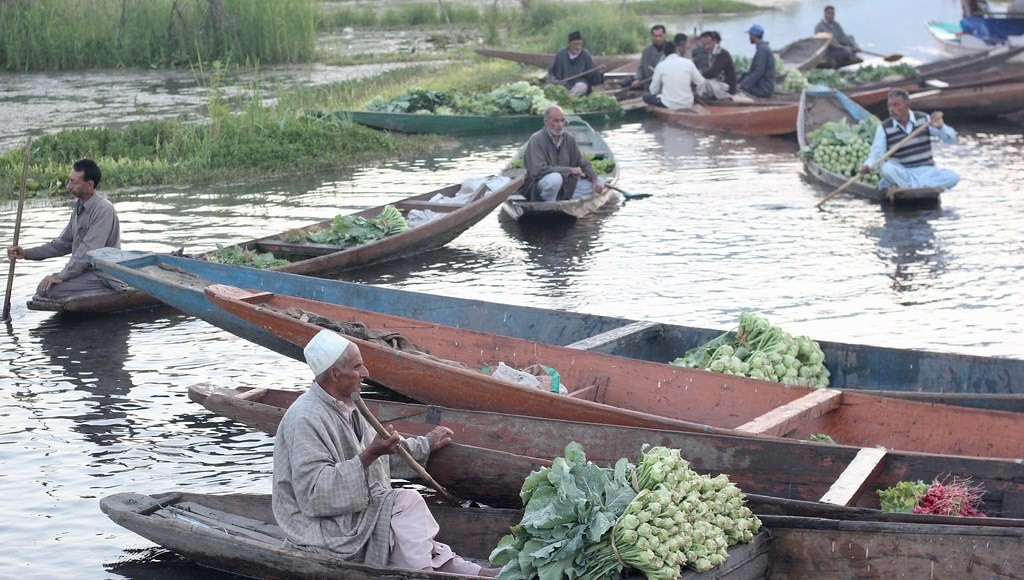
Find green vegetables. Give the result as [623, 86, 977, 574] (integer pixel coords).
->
[365, 81, 621, 115]
[805, 117, 883, 184]
[666, 312, 831, 388]
[731, 53, 920, 94]
[487, 444, 763, 580]
[198, 242, 291, 271]
[308, 204, 408, 248]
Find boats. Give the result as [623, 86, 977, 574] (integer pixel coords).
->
[88, 247, 1024, 413]
[27, 168, 527, 314]
[204, 284, 1024, 458]
[501, 116, 619, 229]
[304, 107, 626, 134]
[475, 0, 1024, 135]
[99, 492, 1024, 580]
[189, 383, 1024, 527]
[796, 86, 959, 202]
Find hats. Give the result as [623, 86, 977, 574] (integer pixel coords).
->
[303, 330, 350, 377]
[744, 24, 764, 37]
[568, 31, 581, 41]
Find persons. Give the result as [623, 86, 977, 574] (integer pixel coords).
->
[862, 89, 961, 189]
[270, 329, 501, 578]
[736, 23, 776, 97]
[517, 106, 603, 201]
[633, 24, 667, 92]
[547, 30, 603, 99]
[641, 33, 704, 109]
[691, 30, 737, 100]
[814, 6, 855, 60]
[7, 159, 122, 297]
